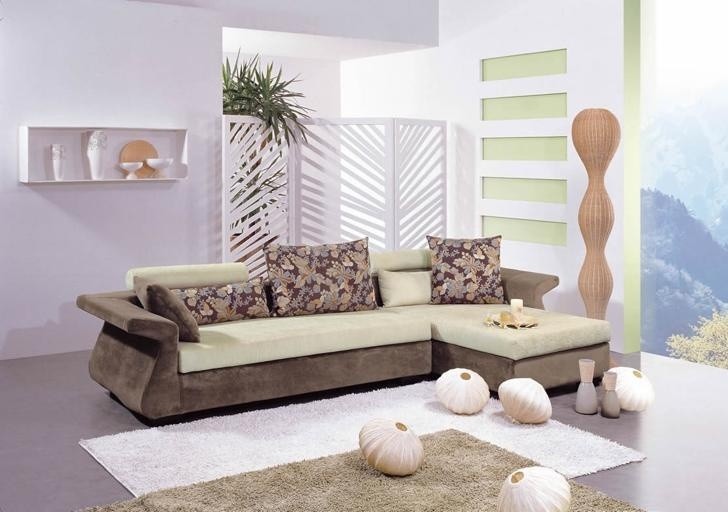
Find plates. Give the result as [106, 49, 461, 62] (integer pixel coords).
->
[121, 140, 158, 175]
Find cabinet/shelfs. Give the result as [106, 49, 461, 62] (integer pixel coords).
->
[19, 124, 192, 185]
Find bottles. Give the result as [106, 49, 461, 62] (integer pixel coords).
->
[510, 298, 524, 321]
[86, 131, 108, 180]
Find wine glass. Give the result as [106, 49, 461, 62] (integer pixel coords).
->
[121, 161, 143, 179]
[146, 159, 174, 179]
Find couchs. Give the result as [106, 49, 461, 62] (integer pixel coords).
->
[75, 251, 433, 427]
[366, 249, 611, 391]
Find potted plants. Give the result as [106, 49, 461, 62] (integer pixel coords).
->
[221, 47, 315, 274]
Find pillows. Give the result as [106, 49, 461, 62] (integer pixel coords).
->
[377, 268, 432, 308]
[133, 276, 200, 344]
[169, 277, 271, 326]
[426, 235, 508, 304]
[263, 236, 379, 317]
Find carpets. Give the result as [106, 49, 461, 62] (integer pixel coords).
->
[68, 429, 640, 512]
[77, 377, 648, 499]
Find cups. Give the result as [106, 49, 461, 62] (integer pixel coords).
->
[51, 143, 64, 181]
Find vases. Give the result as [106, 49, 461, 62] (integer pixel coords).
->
[600, 371, 621, 418]
[575, 359, 598, 415]
